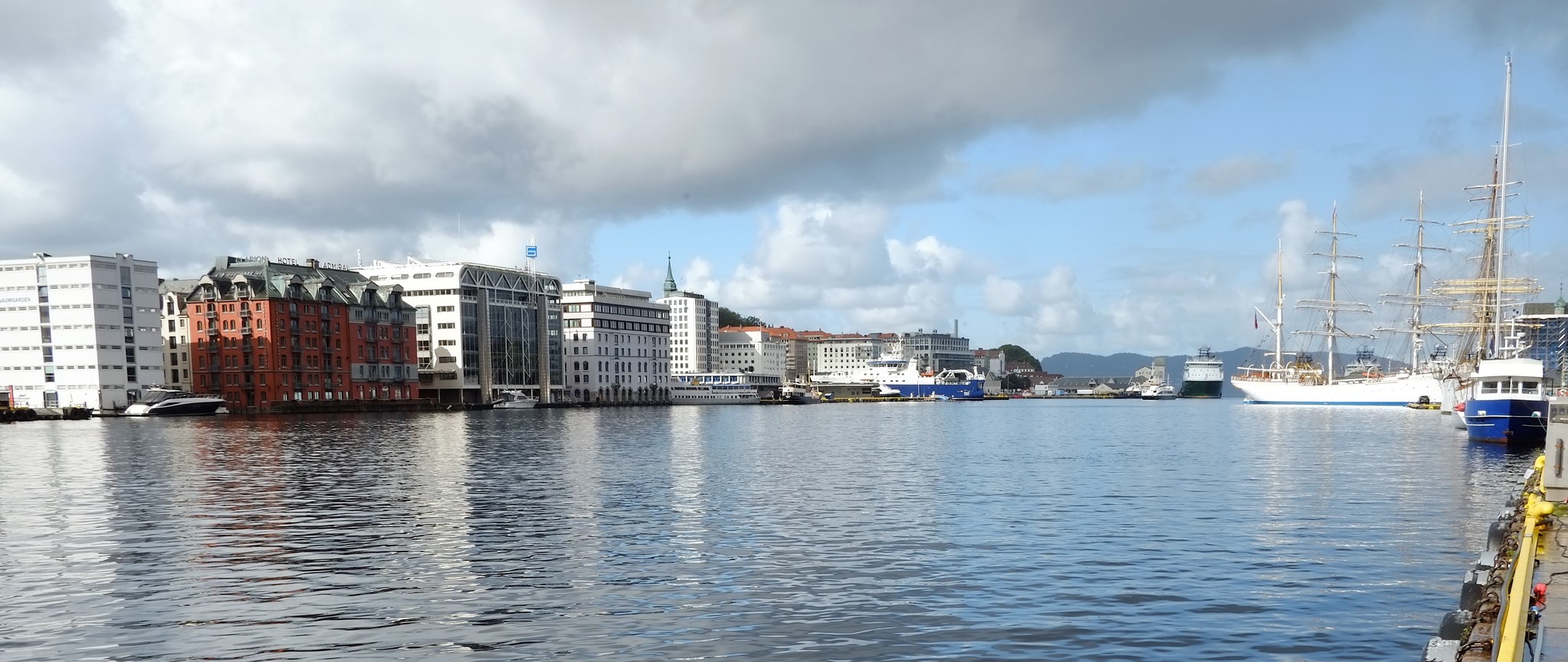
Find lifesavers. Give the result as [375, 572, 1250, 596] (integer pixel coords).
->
[760, 401, 784, 405]
[1560, 389, 1568, 396]
[430, 401, 434, 405]
[821, 401, 839, 403]
[985, 397, 1009, 400]
[840, 399, 886, 402]
[890, 399, 906, 401]
[1421, 476, 1527, 662]
[0, 407, 91, 422]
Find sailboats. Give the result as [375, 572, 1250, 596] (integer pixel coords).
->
[1228, 44, 1557, 445]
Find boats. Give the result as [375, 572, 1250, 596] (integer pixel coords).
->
[116, 380, 230, 417]
[1124, 360, 1178, 400]
[1177, 344, 1225, 399]
[779, 333, 1010, 401]
[490, 386, 540, 409]
[670, 381, 762, 406]
